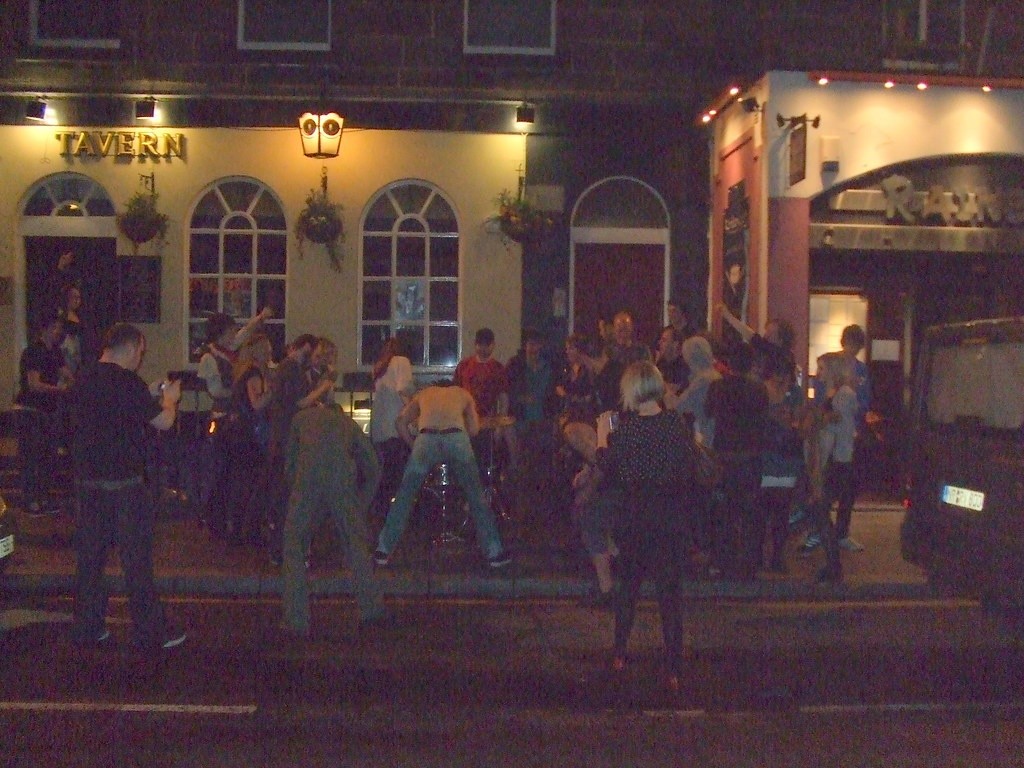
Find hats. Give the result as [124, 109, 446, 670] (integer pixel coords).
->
[475, 328, 496, 344]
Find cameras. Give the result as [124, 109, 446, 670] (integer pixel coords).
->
[160, 383, 167, 390]
[610, 414, 620, 431]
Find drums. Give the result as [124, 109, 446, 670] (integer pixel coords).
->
[425, 464, 459, 487]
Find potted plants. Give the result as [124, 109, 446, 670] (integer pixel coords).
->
[115, 190, 171, 253]
[480, 192, 567, 253]
[294, 190, 347, 272]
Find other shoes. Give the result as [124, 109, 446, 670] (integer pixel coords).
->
[37, 491, 61, 514]
[357, 608, 418, 632]
[654, 664, 684, 693]
[602, 645, 625, 671]
[814, 565, 842, 586]
[267, 611, 312, 638]
[156, 479, 792, 600]
[787, 506, 809, 530]
[16, 491, 42, 519]
[837, 537, 865, 552]
[76, 628, 109, 644]
[142, 628, 187, 649]
[797, 530, 822, 551]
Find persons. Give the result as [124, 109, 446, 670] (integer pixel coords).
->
[370, 339, 415, 505]
[193, 306, 334, 543]
[370, 379, 513, 569]
[70, 321, 186, 650]
[553, 296, 803, 608]
[453, 330, 508, 497]
[280, 402, 387, 633]
[506, 329, 560, 426]
[789, 326, 871, 553]
[598, 361, 691, 680]
[48, 249, 89, 341]
[11, 311, 75, 509]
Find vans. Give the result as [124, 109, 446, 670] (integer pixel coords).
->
[898, 314, 1023, 625]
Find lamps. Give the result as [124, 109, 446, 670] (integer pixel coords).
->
[136, 96, 157, 117]
[26, 96, 47, 120]
[296, 80, 346, 159]
[739, 97, 765, 114]
[515, 90, 535, 123]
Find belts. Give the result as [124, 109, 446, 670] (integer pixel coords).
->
[420, 428, 462, 434]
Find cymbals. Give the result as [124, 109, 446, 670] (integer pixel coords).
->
[482, 415, 517, 430]
